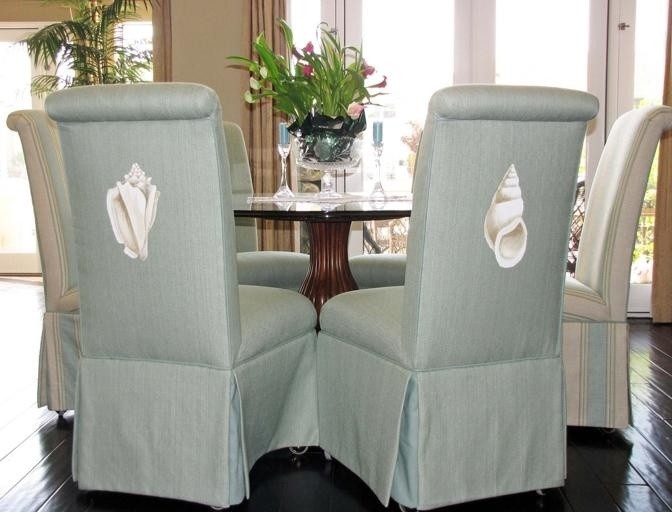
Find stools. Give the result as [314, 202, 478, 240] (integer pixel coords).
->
[348, 253, 407, 292]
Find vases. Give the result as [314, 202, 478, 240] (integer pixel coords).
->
[294, 128, 360, 199]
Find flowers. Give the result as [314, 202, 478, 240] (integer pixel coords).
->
[225, 20, 388, 164]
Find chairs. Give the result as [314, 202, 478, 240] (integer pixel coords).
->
[316, 87, 599, 510]
[7, 110, 82, 428]
[43, 81, 317, 506]
[563, 106, 672, 430]
[221, 121, 314, 296]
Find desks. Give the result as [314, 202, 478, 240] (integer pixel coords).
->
[231, 194, 412, 331]
